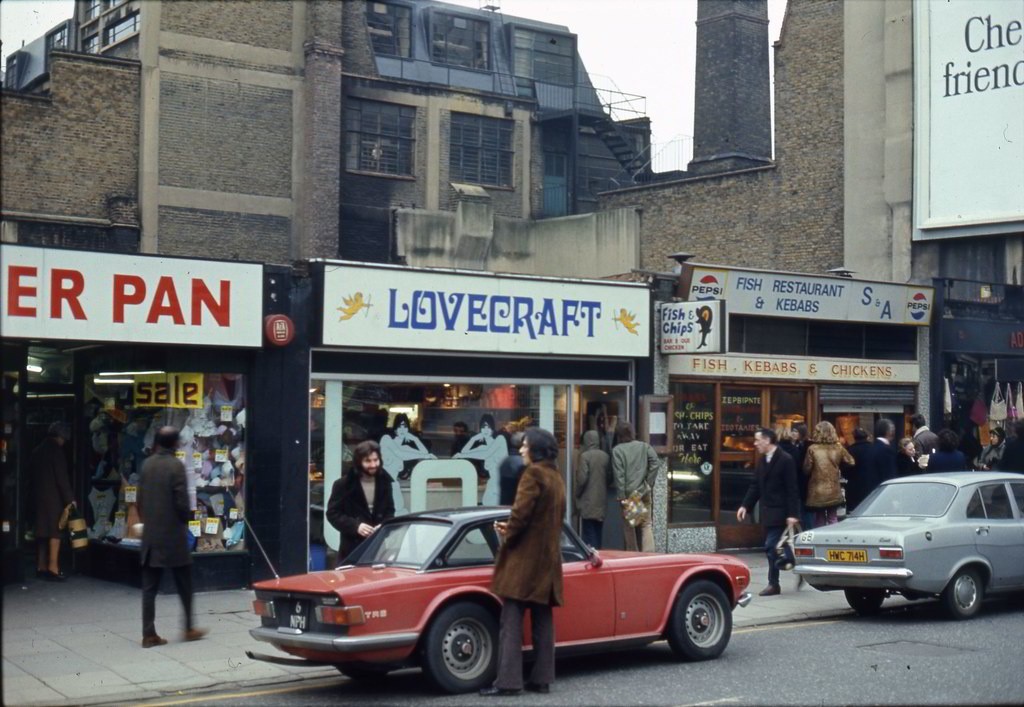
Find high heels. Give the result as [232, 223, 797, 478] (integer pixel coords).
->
[36, 569, 64, 582]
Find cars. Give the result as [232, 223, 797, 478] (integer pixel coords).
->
[244, 501, 756, 695]
[792, 470, 1024, 620]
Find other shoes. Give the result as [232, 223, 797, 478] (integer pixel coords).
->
[479, 686, 524, 697]
[184, 629, 209, 641]
[143, 633, 167, 647]
[523, 680, 550, 694]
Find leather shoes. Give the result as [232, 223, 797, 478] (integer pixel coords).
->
[758, 585, 781, 596]
[797, 574, 803, 591]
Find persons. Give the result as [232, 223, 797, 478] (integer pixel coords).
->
[135, 426, 210, 649]
[327, 439, 395, 569]
[451, 422, 488, 476]
[736, 425, 807, 595]
[499, 430, 528, 506]
[574, 431, 611, 550]
[613, 422, 661, 553]
[479, 427, 566, 695]
[33, 420, 76, 582]
[781, 414, 1024, 530]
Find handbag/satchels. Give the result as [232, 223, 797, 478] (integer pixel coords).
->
[774, 521, 804, 571]
[56, 503, 89, 553]
[990, 382, 1023, 421]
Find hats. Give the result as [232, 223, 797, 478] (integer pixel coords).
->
[989, 427, 1006, 438]
